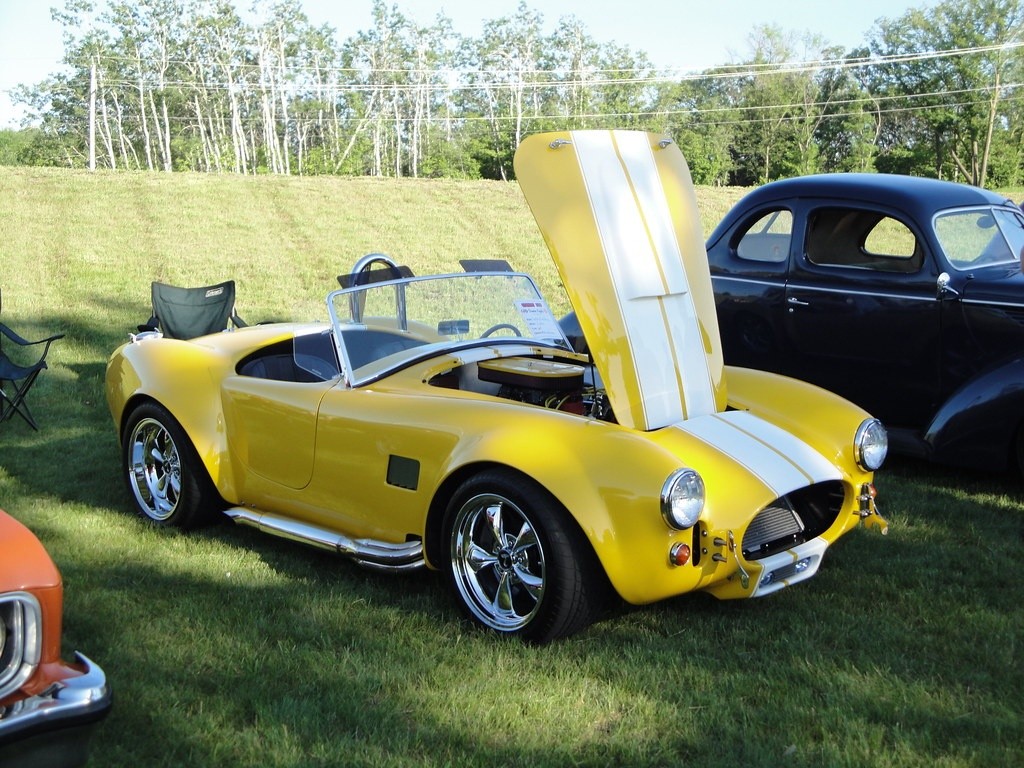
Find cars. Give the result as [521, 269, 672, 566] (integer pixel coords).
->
[559, 174, 1024, 498]
[0, 508, 113, 740]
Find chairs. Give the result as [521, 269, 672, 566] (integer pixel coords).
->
[370, 339, 429, 362]
[136, 281, 272, 340]
[0, 322, 65, 433]
[236, 354, 339, 383]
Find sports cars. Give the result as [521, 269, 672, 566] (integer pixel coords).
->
[105, 130, 889, 651]
